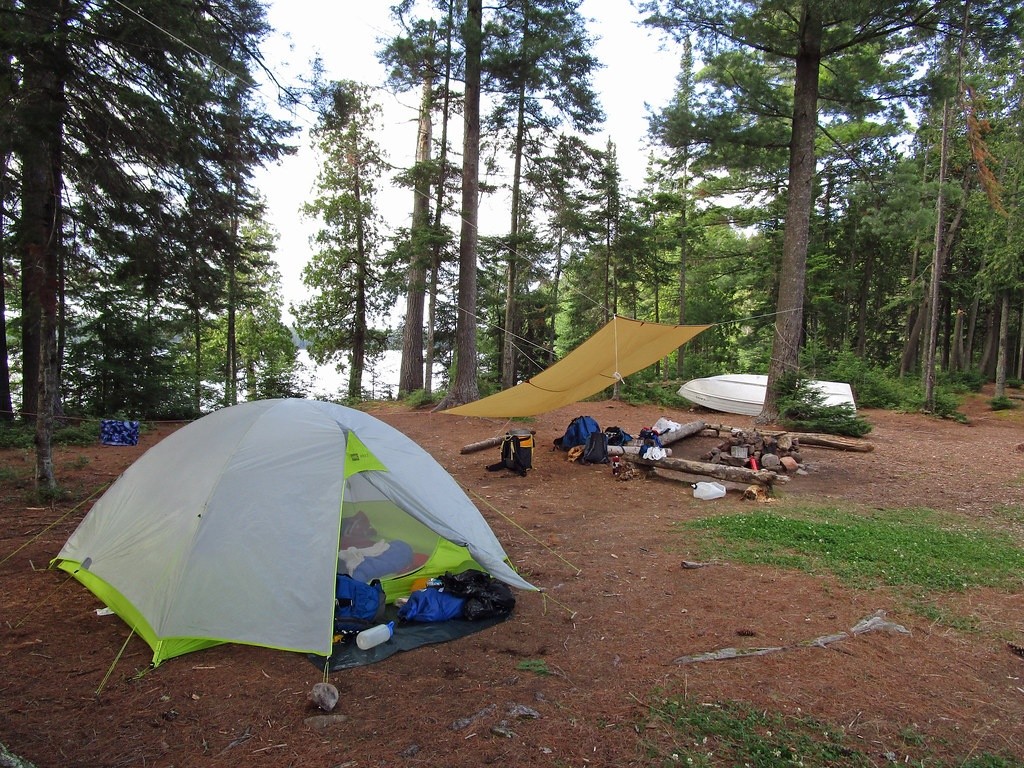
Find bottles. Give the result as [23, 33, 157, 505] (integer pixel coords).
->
[613, 455, 620, 474]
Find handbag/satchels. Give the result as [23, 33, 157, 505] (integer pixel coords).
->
[398, 587, 468, 623]
[438, 569, 516, 620]
[553, 415, 600, 452]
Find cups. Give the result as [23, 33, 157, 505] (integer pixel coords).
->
[356, 621, 395, 650]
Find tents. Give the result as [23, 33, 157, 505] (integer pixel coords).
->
[47, 398, 544, 670]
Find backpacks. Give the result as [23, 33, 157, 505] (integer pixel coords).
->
[486, 433, 535, 477]
[335, 573, 386, 624]
[578, 432, 609, 464]
